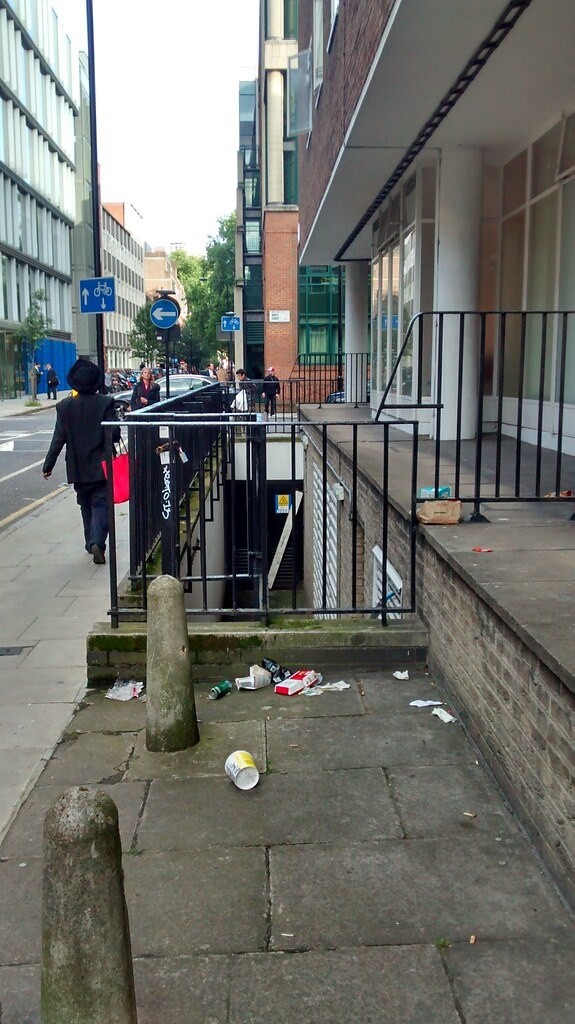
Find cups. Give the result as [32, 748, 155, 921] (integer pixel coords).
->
[224, 750, 259, 790]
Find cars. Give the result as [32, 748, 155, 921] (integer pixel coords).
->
[326, 375, 407, 405]
[107, 372, 244, 424]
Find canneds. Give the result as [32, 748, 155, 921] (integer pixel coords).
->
[207, 680, 232, 700]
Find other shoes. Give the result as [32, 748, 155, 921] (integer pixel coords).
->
[93, 544, 106, 565]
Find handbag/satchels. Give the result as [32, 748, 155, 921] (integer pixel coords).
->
[230, 389, 247, 411]
[50, 369, 59, 386]
[102, 436, 130, 504]
[135, 384, 140, 405]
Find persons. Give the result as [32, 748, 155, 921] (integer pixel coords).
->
[103, 367, 113, 394]
[32, 362, 43, 399]
[42, 358, 122, 564]
[261, 366, 282, 417]
[171, 355, 234, 387]
[130, 367, 160, 415]
[237, 370, 258, 432]
[45, 364, 59, 400]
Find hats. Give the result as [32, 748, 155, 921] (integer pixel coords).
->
[67, 359, 104, 392]
[266, 367, 274, 372]
